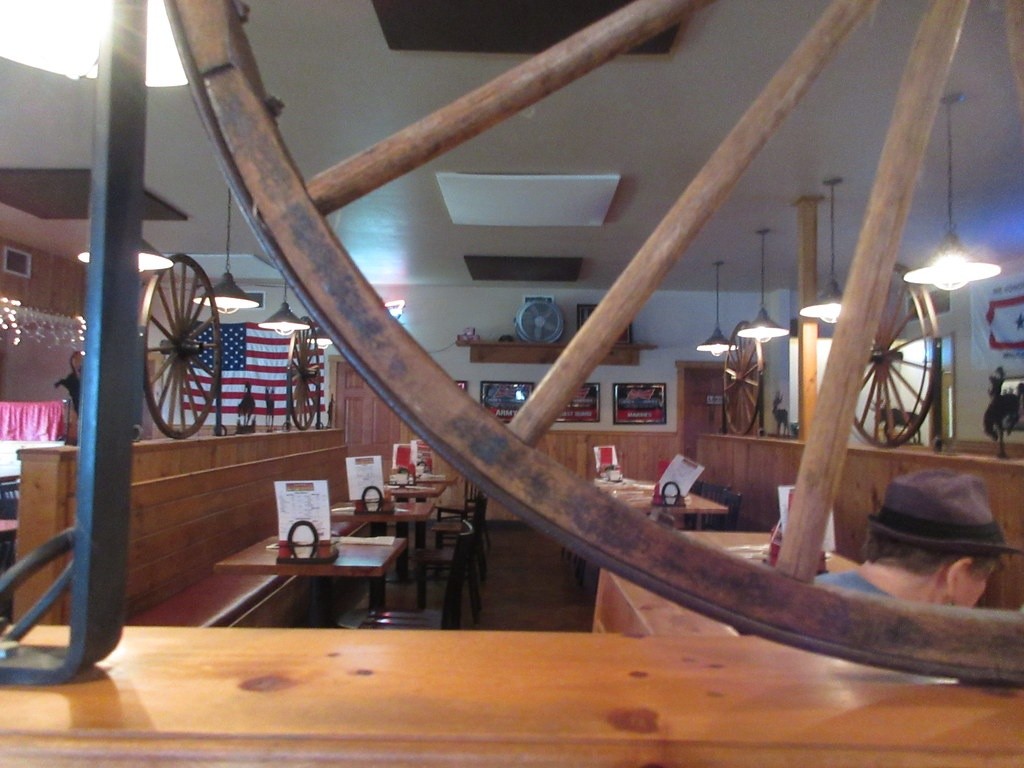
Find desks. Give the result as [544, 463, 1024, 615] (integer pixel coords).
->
[682, 532, 866, 578]
[212, 535, 408, 627]
[591, 477, 729, 516]
[380, 482, 447, 584]
[390, 474, 458, 549]
[329, 501, 435, 613]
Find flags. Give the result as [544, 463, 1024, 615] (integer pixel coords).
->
[180, 321, 327, 416]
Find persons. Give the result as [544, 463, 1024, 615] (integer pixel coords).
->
[811, 468, 1024, 609]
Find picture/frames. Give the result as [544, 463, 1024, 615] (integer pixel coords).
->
[577, 303, 632, 344]
[555, 382, 601, 422]
[479, 380, 534, 424]
[613, 383, 666, 424]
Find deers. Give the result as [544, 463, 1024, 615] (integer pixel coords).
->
[984, 368, 1024, 440]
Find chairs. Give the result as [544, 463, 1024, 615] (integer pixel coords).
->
[677, 479, 742, 534]
[366, 477, 490, 630]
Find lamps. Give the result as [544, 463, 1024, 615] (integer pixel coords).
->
[191, 188, 259, 316]
[77, 235, 175, 272]
[696, 261, 740, 356]
[901, 94, 1003, 291]
[799, 175, 843, 324]
[307, 325, 334, 350]
[737, 228, 790, 343]
[258, 277, 311, 337]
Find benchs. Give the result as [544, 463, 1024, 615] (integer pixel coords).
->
[14, 445, 369, 628]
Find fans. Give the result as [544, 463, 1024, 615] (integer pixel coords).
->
[514, 298, 564, 342]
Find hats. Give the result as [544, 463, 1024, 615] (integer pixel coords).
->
[868, 470, 1024, 554]
